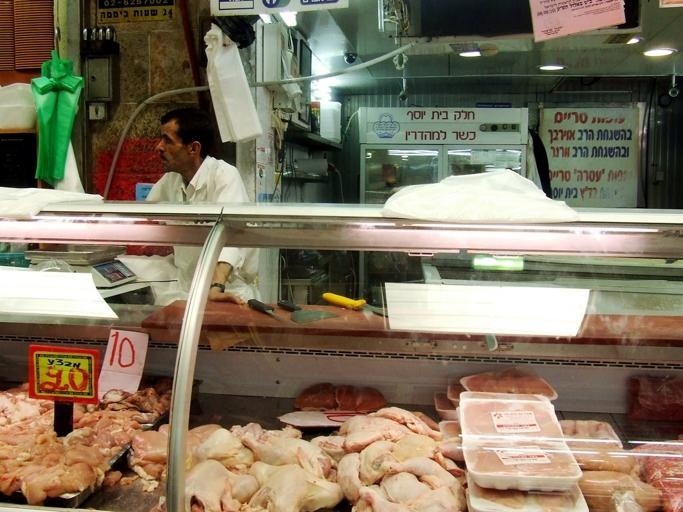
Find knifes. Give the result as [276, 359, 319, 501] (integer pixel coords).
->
[247, 298, 280, 323]
[323, 291, 388, 318]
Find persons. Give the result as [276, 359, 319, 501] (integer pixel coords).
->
[144, 108, 262, 306]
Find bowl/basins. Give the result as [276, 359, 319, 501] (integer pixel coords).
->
[0, 82, 37, 131]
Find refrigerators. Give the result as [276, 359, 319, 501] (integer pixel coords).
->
[360, 107, 527, 320]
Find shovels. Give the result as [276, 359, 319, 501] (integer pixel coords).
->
[277, 299, 338, 323]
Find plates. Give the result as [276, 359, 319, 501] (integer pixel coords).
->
[432, 369, 557, 422]
[278, 409, 368, 427]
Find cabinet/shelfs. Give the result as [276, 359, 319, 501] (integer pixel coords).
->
[281, 130, 344, 184]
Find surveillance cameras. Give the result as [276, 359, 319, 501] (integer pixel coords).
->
[344, 53, 358, 64]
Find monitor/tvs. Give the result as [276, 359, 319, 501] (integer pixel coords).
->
[300, 39, 312, 81]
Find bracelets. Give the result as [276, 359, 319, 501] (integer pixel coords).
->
[209, 283, 225, 293]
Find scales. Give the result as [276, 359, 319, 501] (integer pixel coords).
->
[24, 245, 150, 302]
